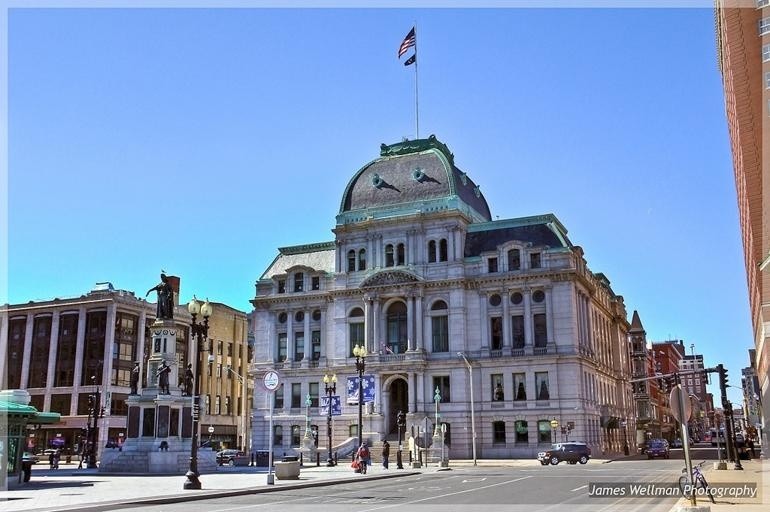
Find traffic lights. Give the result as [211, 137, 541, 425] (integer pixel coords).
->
[631, 381, 645, 393]
[657, 377, 670, 394]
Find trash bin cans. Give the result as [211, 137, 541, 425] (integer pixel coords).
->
[624, 445, 629, 455]
[256, 450, 274, 467]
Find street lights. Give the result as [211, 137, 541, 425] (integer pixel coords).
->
[184, 294, 212, 490]
[353, 343, 367, 447]
[551, 417, 559, 446]
[456, 351, 478, 467]
[323, 371, 337, 467]
[221, 366, 252, 456]
[621, 419, 630, 456]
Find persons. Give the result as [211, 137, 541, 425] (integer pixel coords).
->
[354, 442, 369, 475]
[391, 408, 407, 433]
[144, 274, 176, 323]
[48, 448, 61, 470]
[218, 451, 242, 466]
[744, 433, 756, 458]
[129, 360, 141, 395]
[382, 439, 391, 469]
[182, 362, 196, 394]
[155, 358, 172, 395]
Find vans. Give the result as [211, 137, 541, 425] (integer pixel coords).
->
[710, 428, 726, 446]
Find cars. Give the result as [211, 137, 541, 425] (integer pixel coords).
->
[216, 449, 244, 466]
[671, 437, 694, 448]
[646, 439, 670, 459]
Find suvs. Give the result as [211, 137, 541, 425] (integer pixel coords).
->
[537, 441, 592, 466]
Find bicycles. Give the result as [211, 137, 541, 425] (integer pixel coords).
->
[679, 460, 714, 503]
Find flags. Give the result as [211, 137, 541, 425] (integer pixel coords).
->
[396, 26, 416, 60]
[404, 55, 416, 66]
